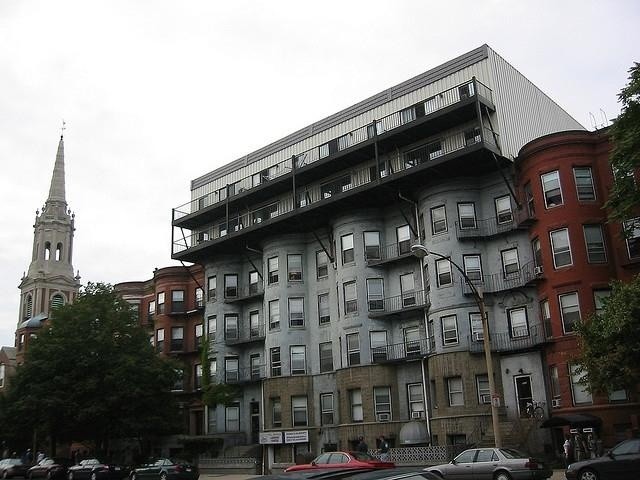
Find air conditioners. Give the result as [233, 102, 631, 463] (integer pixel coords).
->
[411, 411, 421, 420]
[472, 329, 489, 342]
[551, 398, 563, 408]
[533, 265, 544, 275]
[378, 413, 390, 422]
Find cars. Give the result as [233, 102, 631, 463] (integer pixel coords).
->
[564, 438, 640, 480]
[128, 456, 200, 480]
[24, 457, 75, 480]
[284, 450, 395, 473]
[422, 447, 553, 480]
[66, 457, 130, 480]
[243, 465, 446, 480]
[0, 458, 33, 479]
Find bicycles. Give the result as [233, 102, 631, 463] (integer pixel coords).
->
[520, 399, 546, 420]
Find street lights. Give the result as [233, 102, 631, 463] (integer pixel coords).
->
[411, 245, 501, 449]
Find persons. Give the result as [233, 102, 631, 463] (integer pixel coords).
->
[375, 436, 389, 463]
[75, 448, 82, 465]
[357, 436, 368, 454]
[11, 448, 46, 466]
[563, 427, 600, 470]
